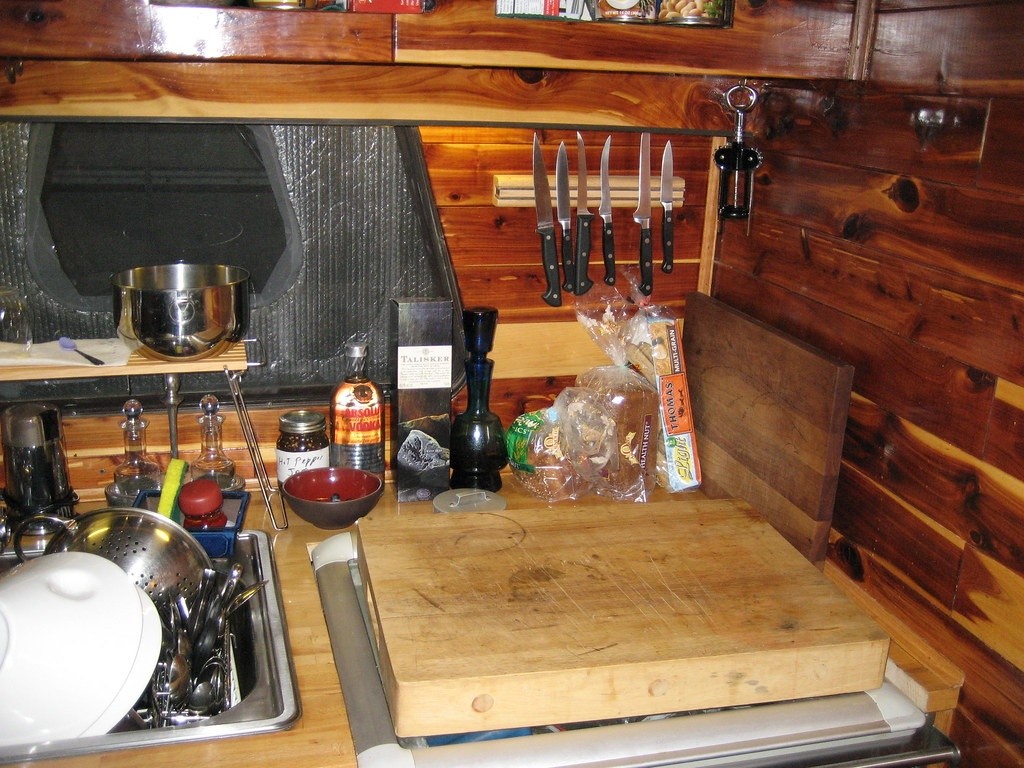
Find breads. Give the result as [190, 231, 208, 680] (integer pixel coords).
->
[509, 336, 667, 499]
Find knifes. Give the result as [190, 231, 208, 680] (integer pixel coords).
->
[531, 132, 674, 308]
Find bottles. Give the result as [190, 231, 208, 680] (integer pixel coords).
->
[449, 305, 508, 493]
[329, 342, 385, 482]
[103, 399, 166, 513]
[189, 394, 245, 491]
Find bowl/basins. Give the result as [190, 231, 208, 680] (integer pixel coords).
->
[108, 262, 250, 361]
[282, 467, 385, 530]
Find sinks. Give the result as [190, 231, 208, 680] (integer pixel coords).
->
[0, 528, 303, 764]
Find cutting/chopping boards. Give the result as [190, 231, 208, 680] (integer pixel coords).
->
[681, 291, 855, 574]
[358, 498, 890, 739]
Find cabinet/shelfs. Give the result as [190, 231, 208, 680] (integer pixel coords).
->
[0, 1, 867, 136]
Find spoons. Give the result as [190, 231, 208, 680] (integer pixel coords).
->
[59, 337, 104, 365]
[167, 563, 271, 718]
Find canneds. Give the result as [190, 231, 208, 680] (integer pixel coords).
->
[276, 409, 331, 493]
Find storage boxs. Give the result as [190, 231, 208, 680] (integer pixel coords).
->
[627, 315, 701, 493]
[390, 296, 453, 502]
